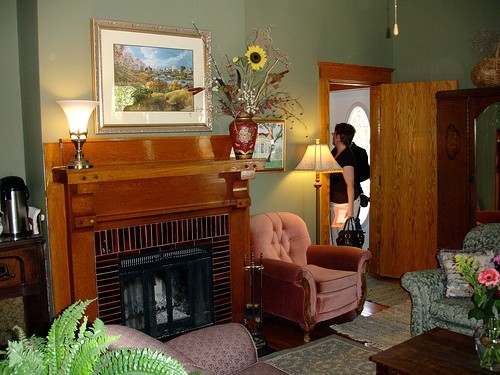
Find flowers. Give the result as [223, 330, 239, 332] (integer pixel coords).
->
[453, 253, 500, 367]
[191, 20, 311, 137]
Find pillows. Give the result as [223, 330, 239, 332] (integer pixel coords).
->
[437, 247, 495, 299]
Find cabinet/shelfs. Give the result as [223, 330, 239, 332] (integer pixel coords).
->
[435, 87, 500, 270]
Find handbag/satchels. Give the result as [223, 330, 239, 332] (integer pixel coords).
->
[336, 217, 365, 248]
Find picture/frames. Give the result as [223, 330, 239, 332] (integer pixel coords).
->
[230, 117, 287, 172]
[90, 17, 213, 134]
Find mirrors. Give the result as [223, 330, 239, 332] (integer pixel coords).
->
[475, 105, 499, 211]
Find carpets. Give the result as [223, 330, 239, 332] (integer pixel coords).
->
[365, 272, 411, 307]
[329, 299, 412, 350]
[258, 334, 384, 374]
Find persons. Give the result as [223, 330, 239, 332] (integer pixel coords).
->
[330, 122, 362, 246]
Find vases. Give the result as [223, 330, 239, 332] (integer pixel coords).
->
[229, 118, 258, 160]
[472, 316, 500, 372]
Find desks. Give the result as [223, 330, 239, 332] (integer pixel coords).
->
[0, 238, 51, 360]
[370, 326, 499, 375]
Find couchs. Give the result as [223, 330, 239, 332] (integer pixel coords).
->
[400, 222, 499, 339]
[102, 323, 290, 375]
[251, 211, 373, 342]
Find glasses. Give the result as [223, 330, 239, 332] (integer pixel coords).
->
[331, 133, 339, 136]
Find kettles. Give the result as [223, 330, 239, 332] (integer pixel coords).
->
[0, 176, 30, 241]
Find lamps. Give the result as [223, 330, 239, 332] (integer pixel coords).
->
[293, 138, 343, 245]
[393, 0, 400, 37]
[57, 100, 97, 169]
